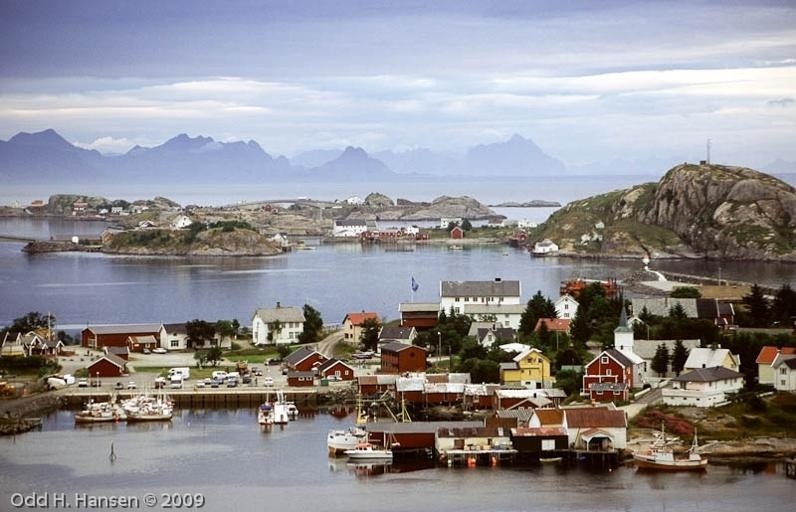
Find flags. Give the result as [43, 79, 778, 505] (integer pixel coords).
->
[411, 276, 419, 293]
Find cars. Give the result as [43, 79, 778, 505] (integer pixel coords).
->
[151, 347, 167, 354]
[76, 356, 285, 388]
[651, 429, 661, 437]
[140, 348, 151, 355]
[349, 347, 381, 361]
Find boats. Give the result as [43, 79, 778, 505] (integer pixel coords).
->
[70, 391, 178, 425]
[252, 386, 395, 468]
[628, 418, 710, 474]
[448, 244, 463, 250]
[294, 238, 316, 250]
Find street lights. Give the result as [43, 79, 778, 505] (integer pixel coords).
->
[717, 266, 722, 287]
[554, 320, 562, 350]
[436, 330, 442, 365]
